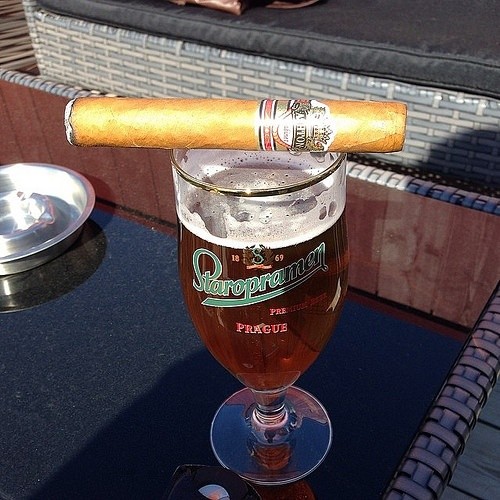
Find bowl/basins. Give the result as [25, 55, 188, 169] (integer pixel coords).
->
[0, 219, 107, 314]
[0, 162, 96, 276]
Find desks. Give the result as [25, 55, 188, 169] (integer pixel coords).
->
[2, 66, 500, 500]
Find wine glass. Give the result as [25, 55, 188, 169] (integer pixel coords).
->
[170, 149, 350, 485]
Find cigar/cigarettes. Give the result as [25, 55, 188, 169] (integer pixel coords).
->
[65, 97, 410, 155]
